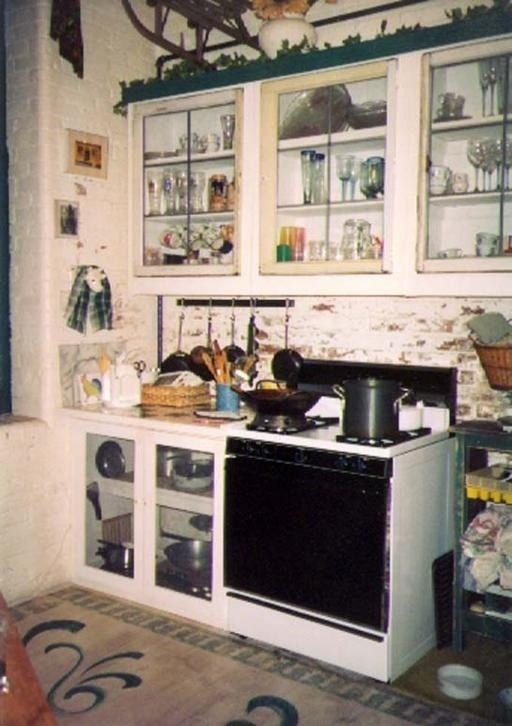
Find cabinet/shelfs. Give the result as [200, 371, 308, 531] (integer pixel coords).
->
[117, 60, 253, 300]
[64, 405, 253, 636]
[404, 5, 512, 299]
[447, 419, 511, 655]
[248, 29, 410, 298]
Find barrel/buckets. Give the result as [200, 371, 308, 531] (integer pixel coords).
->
[70, 356, 102, 408]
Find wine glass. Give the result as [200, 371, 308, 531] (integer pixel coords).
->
[335, 153, 360, 203]
[478, 55, 512, 117]
[467, 132, 512, 192]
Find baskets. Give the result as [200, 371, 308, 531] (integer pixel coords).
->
[473, 338, 512, 391]
[138, 379, 213, 422]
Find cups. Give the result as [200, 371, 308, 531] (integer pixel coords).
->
[361, 155, 385, 199]
[276, 216, 381, 263]
[148, 166, 235, 214]
[431, 165, 469, 195]
[216, 382, 239, 413]
[158, 222, 235, 266]
[436, 92, 465, 118]
[299, 148, 325, 204]
[438, 247, 463, 257]
[144, 113, 234, 157]
[475, 230, 512, 256]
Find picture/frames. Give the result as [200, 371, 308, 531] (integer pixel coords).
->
[52, 197, 87, 242]
[63, 126, 111, 182]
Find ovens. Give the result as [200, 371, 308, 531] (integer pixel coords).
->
[222, 456, 390, 637]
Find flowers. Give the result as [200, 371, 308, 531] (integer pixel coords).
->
[249, 0, 315, 21]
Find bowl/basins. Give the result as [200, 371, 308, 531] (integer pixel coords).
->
[165, 541, 211, 573]
[437, 662, 485, 700]
[345, 108, 386, 126]
[96, 440, 126, 478]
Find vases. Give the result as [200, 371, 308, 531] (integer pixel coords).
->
[252, 9, 320, 62]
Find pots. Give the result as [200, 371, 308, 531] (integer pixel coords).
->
[335, 373, 413, 439]
[92, 537, 133, 569]
[156, 445, 193, 478]
[170, 461, 213, 489]
[240, 379, 322, 414]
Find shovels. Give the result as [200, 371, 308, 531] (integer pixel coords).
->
[87, 482, 102, 521]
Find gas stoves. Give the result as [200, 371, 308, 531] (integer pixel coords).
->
[224, 397, 455, 479]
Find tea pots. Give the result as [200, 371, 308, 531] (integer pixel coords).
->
[100, 362, 142, 407]
